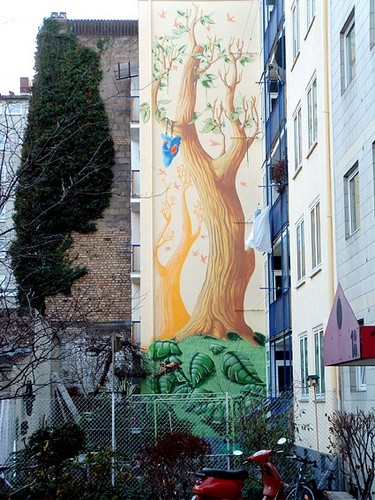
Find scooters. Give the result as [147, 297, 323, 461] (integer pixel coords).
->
[188, 437, 288, 500]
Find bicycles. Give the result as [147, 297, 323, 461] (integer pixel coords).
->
[285, 453, 317, 500]
